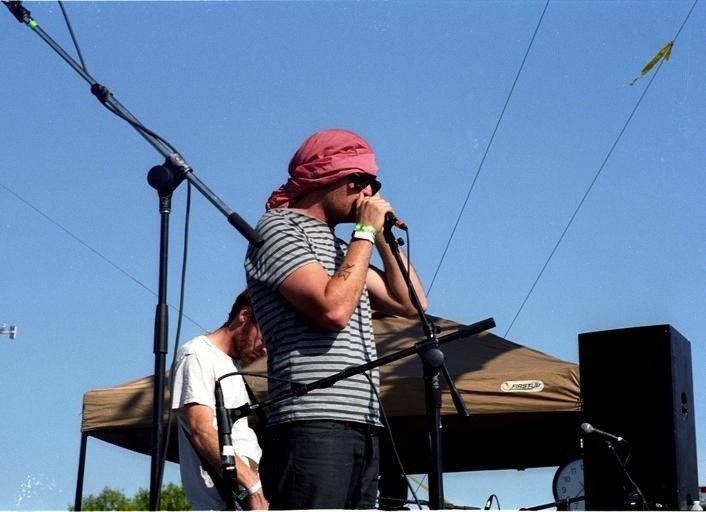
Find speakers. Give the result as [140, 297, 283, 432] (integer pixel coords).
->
[577, 324, 699, 511]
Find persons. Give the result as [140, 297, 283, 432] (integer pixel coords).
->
[171, 287, 271, 511]
[244, 131, 428, 510]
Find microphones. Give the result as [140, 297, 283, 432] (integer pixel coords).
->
[384, 208, 408, 231]
[214, 381, 235, 472]
[579, 422, 622, 443]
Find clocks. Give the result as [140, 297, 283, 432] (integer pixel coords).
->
[552, 457, 586, 511]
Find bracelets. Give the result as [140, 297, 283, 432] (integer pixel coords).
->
[352, 223, 378, 244]
[247, 480, 262, 495]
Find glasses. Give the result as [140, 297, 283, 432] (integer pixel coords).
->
[347, 171, 382, 196]
